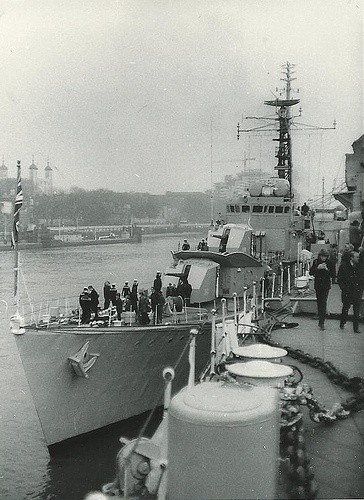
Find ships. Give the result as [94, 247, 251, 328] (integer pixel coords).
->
[0, 188, 142, 250]
[86, 136, 363, 500]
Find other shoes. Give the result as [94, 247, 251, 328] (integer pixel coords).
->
[353, 323, 362, 333]
[340, 321, 344, 328]
[318, 324, 325, 330]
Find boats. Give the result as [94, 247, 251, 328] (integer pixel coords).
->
[10, 61, 337, 455]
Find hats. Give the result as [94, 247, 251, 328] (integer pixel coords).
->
[318, 249, 329, 256]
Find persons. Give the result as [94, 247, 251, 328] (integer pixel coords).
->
[79, 287, 90, 324]
[154, 271, 162, 291]
[132, 279, 139, 301]
[197, 239, 208, 251]
[115, 293, 122, 321]
[173, 284, 176, 291]
[260, 275, 268, 292]
[277, 267, 281, 276]
[338, 252, 362, 333]
[166, 283, 174, 295]
[301, 202, 309, 215]
[104, 280, 111, 309]
[151, 287, 163, 325]
[283, 267, 288, 283]
[139, 290, 150, 325]
[310, 249, 336, 330]
[109, 285, 117, 306]
[185, 280, 192, 306]
[122, 281, 130, 297]
[88, 286, 99, 320]
[349, 220, 362, 247]
[182, 239, 190, 250]
